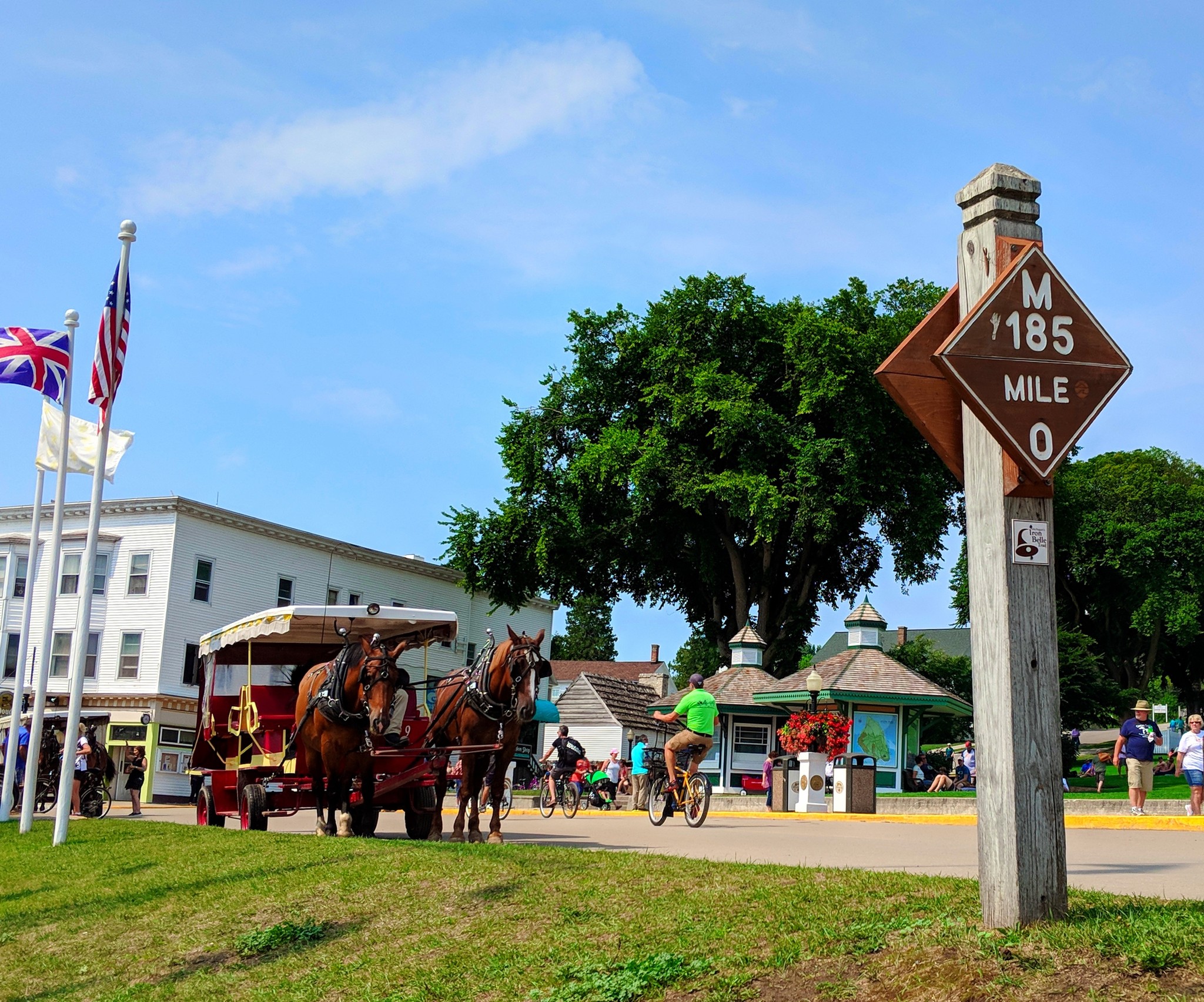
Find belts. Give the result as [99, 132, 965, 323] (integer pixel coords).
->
[687, 728, 712, 738]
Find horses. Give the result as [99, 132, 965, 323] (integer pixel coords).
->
[421, 624, 545, 845]
[295, 636, 415, 837]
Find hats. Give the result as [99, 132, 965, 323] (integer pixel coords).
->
[688, 674, 703, 684]
[957, 756, 963, 761]
[1130, 700, 1152, 710]
[1167, 757, 1175, 761]
[947, 743, 950, 746]
[610, 748, 620, 755]
[965, 741, 971, 747]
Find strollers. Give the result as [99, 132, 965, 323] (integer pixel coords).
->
[580, 770, 613, 811]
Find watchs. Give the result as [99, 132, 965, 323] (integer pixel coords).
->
[134, 766, 138, 768]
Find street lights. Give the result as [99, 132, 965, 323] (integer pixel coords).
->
[627, 728, 635, 761]
[21, 680, 33, 714]
[806, 664, 822, 752]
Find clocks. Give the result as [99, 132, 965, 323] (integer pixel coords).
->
[141, 714, 151, 725]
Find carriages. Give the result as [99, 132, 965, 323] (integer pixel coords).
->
[185, 605, 545, 845]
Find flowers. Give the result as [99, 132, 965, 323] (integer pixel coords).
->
[776, 708, 853, 762]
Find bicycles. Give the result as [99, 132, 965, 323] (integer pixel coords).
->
[21, 750, 112, 819]
[464, 767, 515, 821]
[539, 760, 579, 819]
[642, 715, 712, 828]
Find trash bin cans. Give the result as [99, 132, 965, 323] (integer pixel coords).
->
[770, 755, 799, 812]
[833, 753, 877, 814]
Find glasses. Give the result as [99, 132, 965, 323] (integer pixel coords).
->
[922, 757, 927, 759]
[1189, 721, 1200, 724]
[557, 731, 560, 733]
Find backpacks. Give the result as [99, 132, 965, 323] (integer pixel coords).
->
[561, 736, 583, 763]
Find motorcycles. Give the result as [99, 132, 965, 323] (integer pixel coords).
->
[0, 709, 112, 817]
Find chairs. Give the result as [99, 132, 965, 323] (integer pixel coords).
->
[902, 769, 928, 792]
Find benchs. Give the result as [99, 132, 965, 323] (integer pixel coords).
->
[202, 684, 302, 768]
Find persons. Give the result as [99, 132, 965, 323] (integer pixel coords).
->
[1173, 715, 1204, 815]
[912, 740, 976, 792]
[190, 773, 205, 806]
[1062, 778, 1069, 793]
[124, 737, 147, 817]
[1152, 715, 1191, 776]
[360, 627, 410, 743]
[1071, 726, 1082, 751]
[479, 753, 496, 813]
[653, 674, 720, 818]
[1113, 700, 1164, 816]
[1, 717, 92, 818]
[759, 751, 779, 812]
[825, 755, 834, 786]
[446, 754, 466, 808]
[536, 725, 652, 812]
[1079, 749, 1109, 793]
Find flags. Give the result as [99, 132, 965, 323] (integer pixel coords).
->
[85, 251, 131, 435]
[0, 326, 72, 406]
[36, 399, 136, 483]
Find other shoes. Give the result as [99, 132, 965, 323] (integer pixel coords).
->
[546, 800, 556, 807]
[615, 805, 623, 810]
[638, 808, 648, 811]
[605, 799, 612, 802]
[382, 736, 409, 747]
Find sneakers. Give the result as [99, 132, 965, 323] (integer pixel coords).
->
[1185, 804, 1193, 816]
[1131, 809, 1146, 816]
[689, 804, 699, 819]
[128, 812, 142, 815]
[664, 780, 679, 792]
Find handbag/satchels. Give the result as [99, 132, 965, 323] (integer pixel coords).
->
[1099, 753, 1111, 763]
